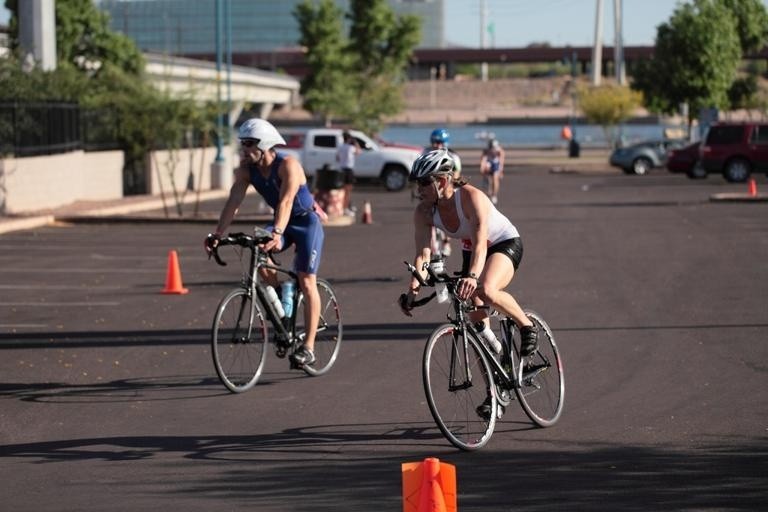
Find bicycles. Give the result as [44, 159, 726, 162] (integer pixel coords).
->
[202, 232, 345, 395]
[397, 257, 567, 449]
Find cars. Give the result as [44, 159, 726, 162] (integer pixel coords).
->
[609, 139, 686, 176]
[666, 141, 707, 178]
[271, 124, 428, 151]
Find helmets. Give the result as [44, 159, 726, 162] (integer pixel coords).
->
[430, 129, 449, 143]
[239, 118, 286, 151]
[412, 150, 456, 178]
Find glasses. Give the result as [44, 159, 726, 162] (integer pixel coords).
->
[240, 140, 255, 148]
[414, 179, 436, 187]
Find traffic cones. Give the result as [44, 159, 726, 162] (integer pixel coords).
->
[747, 176, 758, 196]
[417, 458, 448, 512]
[159, 247, 189, 297]
[361, 199, 373, 224]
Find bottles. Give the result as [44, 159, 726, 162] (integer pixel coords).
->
[475, 322, 503, 354]
[264, 278, 295, 319]
[428, 255, 451, 306]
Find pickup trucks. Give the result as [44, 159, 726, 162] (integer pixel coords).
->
[262, 128, 424, 192]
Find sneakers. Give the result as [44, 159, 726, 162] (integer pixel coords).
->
[476, 397, 505, 419]
[520, 320, 539, 357]
[288, 345, 319, 365]
[442, 246, 451, 257]
[344, 208, 355, 217]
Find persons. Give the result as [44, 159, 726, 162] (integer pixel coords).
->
[409, 129, 462, 255]
[336, 131, 362, 211]
[480, 139, 506, 205]
[398, 147, 540, 418]
[204, 118, 325, 364]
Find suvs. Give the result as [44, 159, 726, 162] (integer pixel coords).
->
[700, 119, 768, 183]
